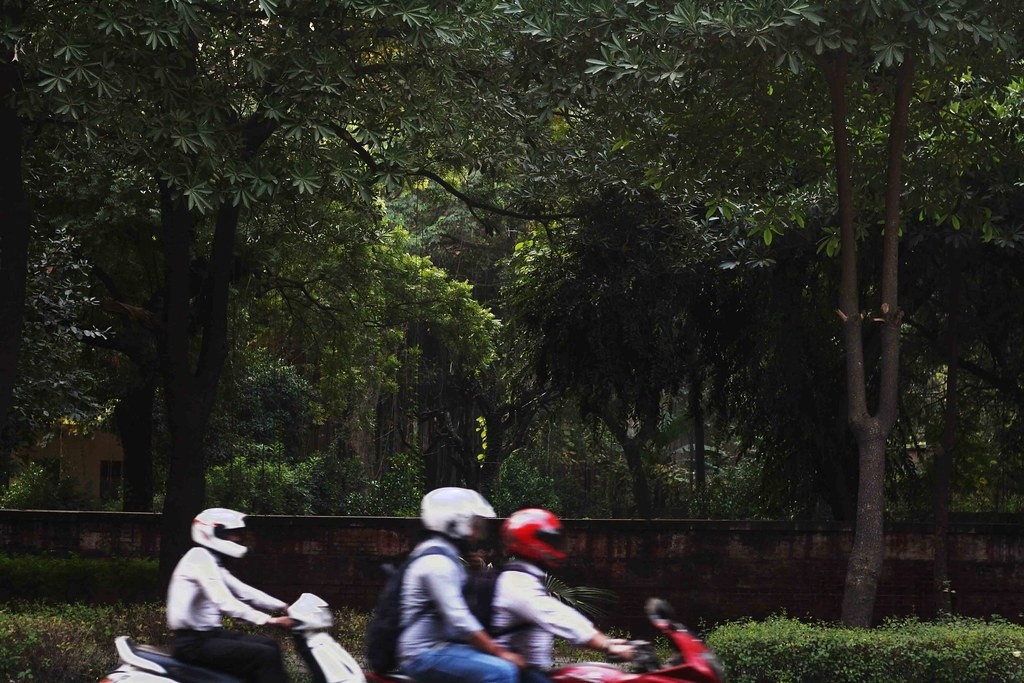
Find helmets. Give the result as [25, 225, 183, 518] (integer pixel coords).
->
[499, 509, 567, 567]
[190, 506, 249, 559]
[420, 485, 497, 541]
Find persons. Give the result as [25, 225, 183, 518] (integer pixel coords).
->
[489, 508, 637, 683]
[397, 487, 527, 683]
[167, 508, 295, 683]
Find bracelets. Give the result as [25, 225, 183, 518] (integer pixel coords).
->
[601, 640, 613, 652]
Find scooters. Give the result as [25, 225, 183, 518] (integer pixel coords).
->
[363, 596, 729, 683]
[98, 593, 368, 683]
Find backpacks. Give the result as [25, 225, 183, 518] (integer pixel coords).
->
[367, 547, 458, 673]
[476, 566, 546, 637]
